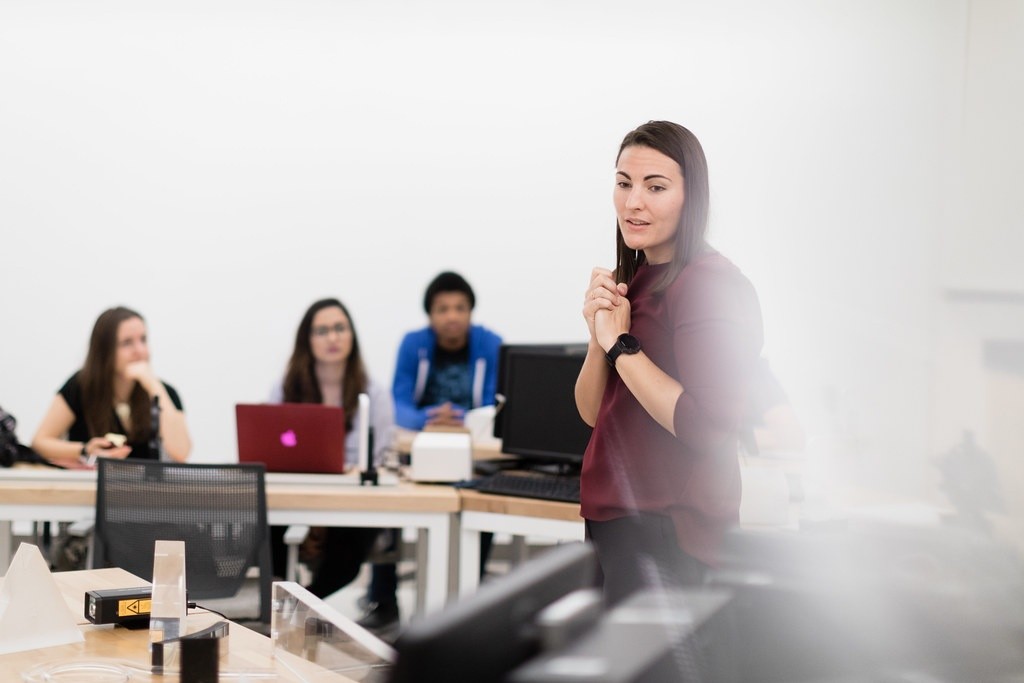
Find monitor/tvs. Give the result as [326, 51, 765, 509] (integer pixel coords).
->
[494, 346, 604, 477]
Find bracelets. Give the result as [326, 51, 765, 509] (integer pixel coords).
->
[79, 444, 88, 465]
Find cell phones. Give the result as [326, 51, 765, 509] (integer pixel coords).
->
[100, 432, 128, 450]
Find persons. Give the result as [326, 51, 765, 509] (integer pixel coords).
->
[267, 299, 392, 600]
[574, 119, 763, 610]
[0, 403, 17, 575]
[356, 271, 503, 632]
[32, 308, 191, 568]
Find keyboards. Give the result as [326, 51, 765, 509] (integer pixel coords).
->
[476, 475, 581, 504]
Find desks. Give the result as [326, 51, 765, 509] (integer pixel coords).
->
[0, 460, 587, 683]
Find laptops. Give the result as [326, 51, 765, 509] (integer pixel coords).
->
[236, 404, 353, 475]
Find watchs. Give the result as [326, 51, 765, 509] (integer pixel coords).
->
[605, 333, 642, 369]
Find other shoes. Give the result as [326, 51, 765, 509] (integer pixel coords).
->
[355, 602, 401, 633]
